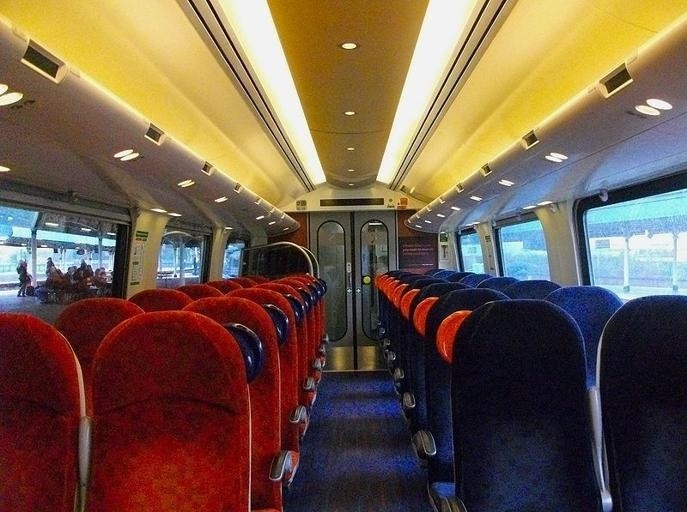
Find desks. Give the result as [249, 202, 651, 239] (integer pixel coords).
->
[156, 271, 174, 288]
[106, 281, 112, 297]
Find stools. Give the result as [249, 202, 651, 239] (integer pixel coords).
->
[36, 280, 100, 306]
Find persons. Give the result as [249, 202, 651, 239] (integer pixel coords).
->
[17, 257, 109, 303]
